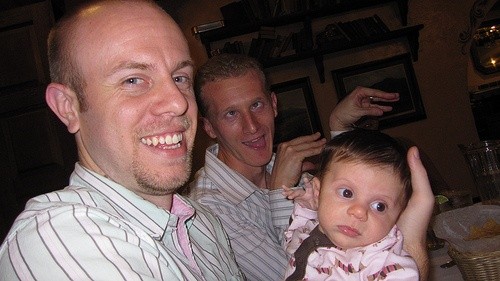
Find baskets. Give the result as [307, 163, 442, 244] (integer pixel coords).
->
[448, 245, 499, 281]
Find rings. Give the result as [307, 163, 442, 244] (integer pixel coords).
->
[369, 96, 374, 103]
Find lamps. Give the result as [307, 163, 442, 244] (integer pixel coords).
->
[459, 0, 500, 74]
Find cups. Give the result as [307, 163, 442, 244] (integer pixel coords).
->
[462, 140, 500, 201]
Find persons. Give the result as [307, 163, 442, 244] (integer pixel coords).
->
[181, 53, 400, 281]
[0, 0, 437, 281]
[280, 126, 420, 281]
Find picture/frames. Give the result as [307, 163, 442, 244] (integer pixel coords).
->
[330, 54, 427, 131]
[258, 75, 325, 155]
